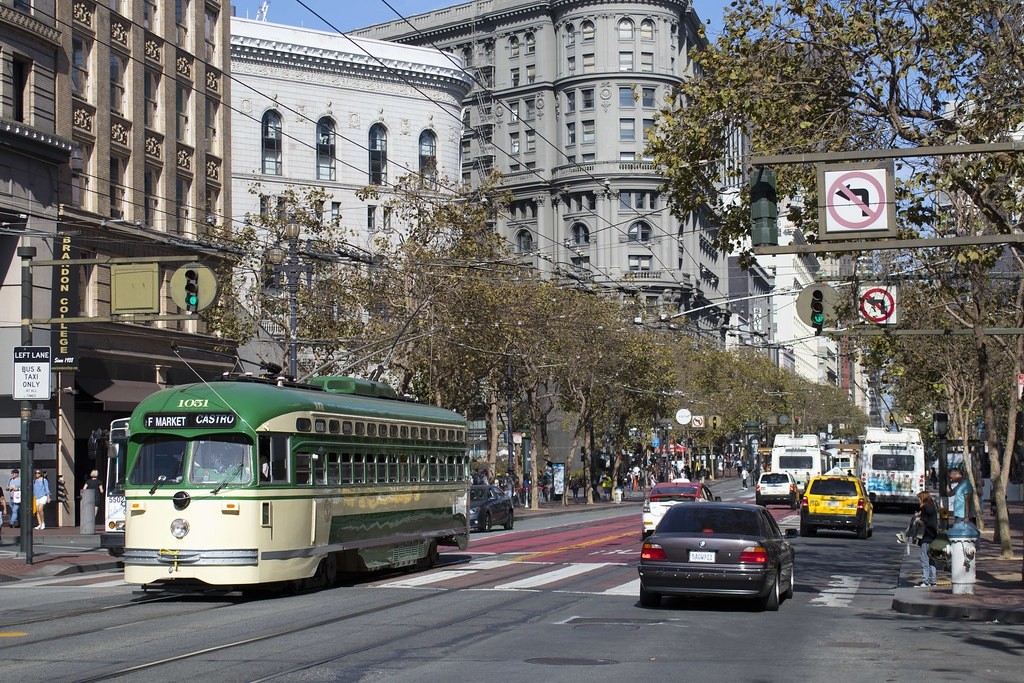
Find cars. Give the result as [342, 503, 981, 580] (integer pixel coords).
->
[470, 484, 514, 532]
[637, 502, 798, 612]
[799, 475, 876, 540]
[641, 482, 722, 540]
[756, 471, 801, 510]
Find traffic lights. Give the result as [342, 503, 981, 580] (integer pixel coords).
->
[886, 411, 898, 424]
[21, 420, 47, 443]
[169, 262, 218, 313]
[708, 415, 721, 429]
[796, 283, 842, 328]
[795, 416, 802, 425]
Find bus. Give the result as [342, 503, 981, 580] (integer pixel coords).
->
[825, 440, 862, 478]
[771, 434, 835, 495]
[856, 427, 925, 505]
[106, 373, 470, 590]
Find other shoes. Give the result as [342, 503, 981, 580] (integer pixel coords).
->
[929, 582, 937, 587]
[9, 524, 13, 528]
[914, 580, 931, 587]
[34, 524, 41, 529]
[41, 523, 46, 529]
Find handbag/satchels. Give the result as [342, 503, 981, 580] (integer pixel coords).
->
[12, 487, 21, 503]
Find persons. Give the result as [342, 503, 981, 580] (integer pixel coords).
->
[239, 452, 269, 481]
[32, 469, 51, 530]
[0, 486, 6, 540]
[470, 452, 750, 507]
[929, 467, 938, 489]
[5, 469, 21, 528]
[83, 469, 104, 518]
[979, 472, 986, 517]
[913, 491, 938, 587]
[947, 466, 977, 525]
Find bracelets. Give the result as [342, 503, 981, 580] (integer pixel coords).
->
[965, 516, 970, 518]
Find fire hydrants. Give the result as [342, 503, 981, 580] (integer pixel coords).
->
[942, 521, 981, 595]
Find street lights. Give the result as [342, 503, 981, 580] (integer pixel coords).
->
[933, 412, 949, 530]
[270, 214, 317, 381]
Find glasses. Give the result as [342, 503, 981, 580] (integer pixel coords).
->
[35, 473, 40, 474]
